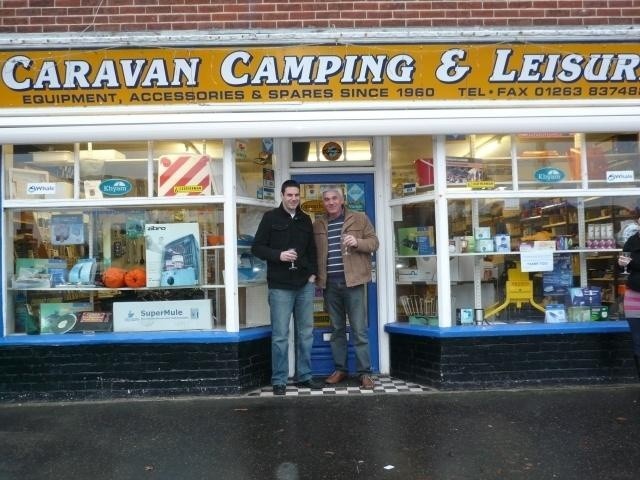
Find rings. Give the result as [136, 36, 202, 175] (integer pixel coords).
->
[350, 240, 354, 244]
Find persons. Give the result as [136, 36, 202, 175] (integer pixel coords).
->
[485, 253, 510, 288]
[519, 226, 559, 243]
[251, 179, 324, 396]
[311, 184, 382, 390]
[618, 229, 640, 375]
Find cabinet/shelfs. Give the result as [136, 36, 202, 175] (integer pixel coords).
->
[2, 196, 277, 331]
[390, 187, 640, 328]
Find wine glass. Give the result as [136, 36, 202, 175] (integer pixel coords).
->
[288, 248, 298, 270]
[618, 250, 634, 277]
[341, 234, 351, 256]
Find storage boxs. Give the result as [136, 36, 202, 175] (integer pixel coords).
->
[159, 155, 211, 197]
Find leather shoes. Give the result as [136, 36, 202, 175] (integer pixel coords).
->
[357, 373, 374, 390]
[296, 379, 326, 389]
[273, 383, 286, 395]
[327, 368, 349, 383]
